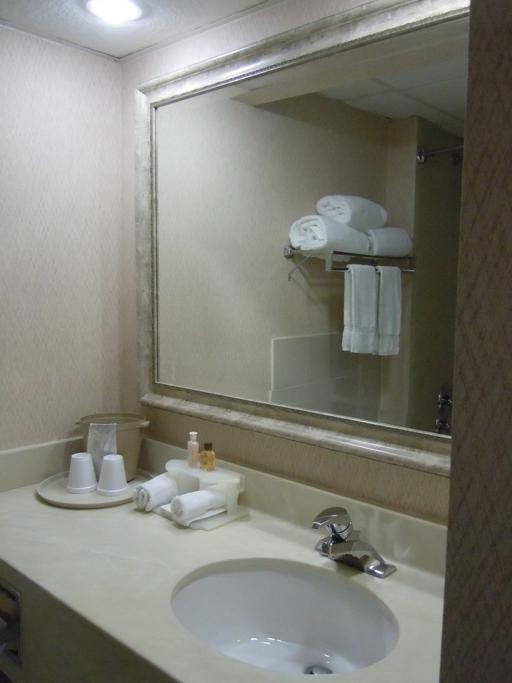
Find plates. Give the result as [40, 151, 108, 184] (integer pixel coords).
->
[35, 464, 155, 509]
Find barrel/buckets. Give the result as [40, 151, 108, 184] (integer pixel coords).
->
[75, 413, 150, 484]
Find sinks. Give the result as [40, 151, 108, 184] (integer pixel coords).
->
[170, 558, 399, 675]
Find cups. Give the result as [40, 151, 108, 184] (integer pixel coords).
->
[67, 452, 129, 497]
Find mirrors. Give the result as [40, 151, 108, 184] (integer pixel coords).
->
[130, 0, 469, 477]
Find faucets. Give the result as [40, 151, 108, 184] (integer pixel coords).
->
[311, 505, 398, 579]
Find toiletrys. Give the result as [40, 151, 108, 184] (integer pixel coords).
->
[187, 432, 215, 472]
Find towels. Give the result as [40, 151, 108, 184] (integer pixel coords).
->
[137, 474, 231, 530]
[285, 183, 413, 359]
